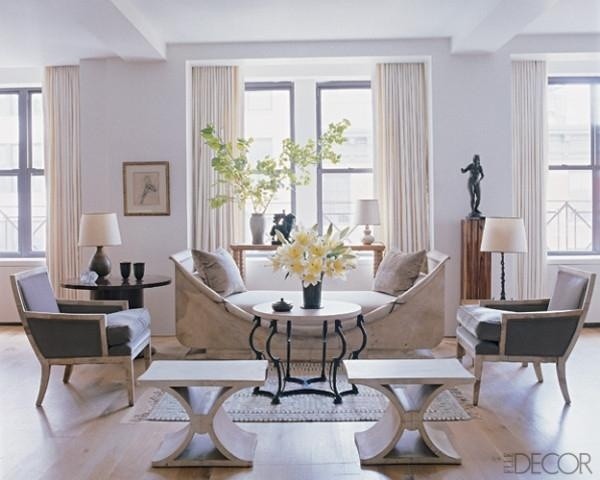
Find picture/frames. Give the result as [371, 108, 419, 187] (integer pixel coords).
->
[122, 160, 173, 217]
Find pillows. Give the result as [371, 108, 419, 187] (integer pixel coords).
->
[370, 247, 430, 299]
[190, 245, 248, 297]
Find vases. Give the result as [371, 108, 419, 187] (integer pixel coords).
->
[296, 270, 326, 309]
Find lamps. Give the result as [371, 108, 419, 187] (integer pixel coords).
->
[479, 216, 531, 302]
[355, 200, 385, 246]
[77, 212, 122, 284]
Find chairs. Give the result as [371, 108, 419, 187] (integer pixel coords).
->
[9, 265, 153, 407]
[454, 264, 597, 406]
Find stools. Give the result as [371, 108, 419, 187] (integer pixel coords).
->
[342, 358, 479, 465]
[135, 359, 270, 468]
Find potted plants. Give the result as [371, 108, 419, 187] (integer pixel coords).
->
[201, 116, 354, 245]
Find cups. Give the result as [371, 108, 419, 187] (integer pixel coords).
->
[120, 262, 131, 280]
[133, 262, 145, 282]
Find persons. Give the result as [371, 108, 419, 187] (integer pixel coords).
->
[461, 155, 485, 216]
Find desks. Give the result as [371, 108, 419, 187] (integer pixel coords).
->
[59, 274, 172, 358]
[226, 243, 386, 287]
[247, 299, 368, 405]
[457, 298, 530, 368]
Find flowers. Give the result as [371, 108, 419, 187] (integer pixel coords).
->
[264, 221, 360, 289]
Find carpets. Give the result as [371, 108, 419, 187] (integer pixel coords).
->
[129, 361, 479, 420]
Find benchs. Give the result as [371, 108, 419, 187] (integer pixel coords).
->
[169, 248, 452, 360]
